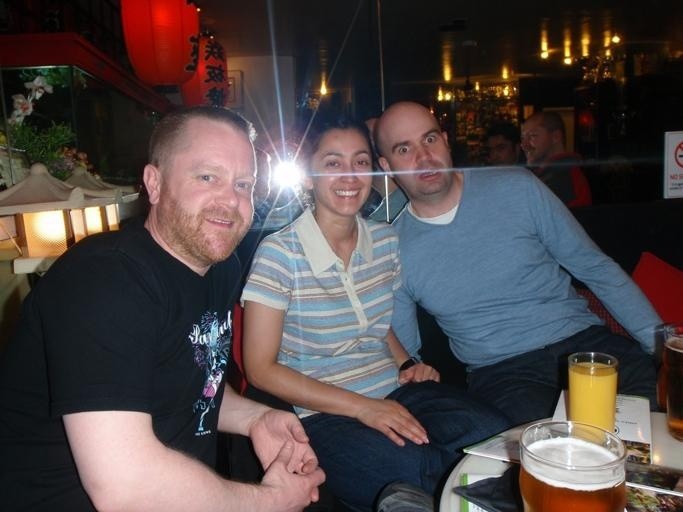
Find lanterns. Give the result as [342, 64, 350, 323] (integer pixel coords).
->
[120, 0, 200, 94]
[180, 36, 229, 106]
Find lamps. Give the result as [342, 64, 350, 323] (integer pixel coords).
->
[0, 163, 140, 275]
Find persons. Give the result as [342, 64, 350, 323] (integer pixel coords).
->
[238, 113, 516, 512]
[0, 102, 330, 512]
[520, 110, 599, 245]
[373, 98, 675, 425]
[482, 116, 527, 166]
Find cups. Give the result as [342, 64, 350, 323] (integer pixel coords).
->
[515, 418, 630, 512]
[662, 321, 683, 443]
[566, 350, 620, 444]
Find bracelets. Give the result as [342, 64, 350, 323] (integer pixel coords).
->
[400, 356, 420, 371]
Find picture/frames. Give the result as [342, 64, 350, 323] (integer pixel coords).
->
[222, 71, 242, 109]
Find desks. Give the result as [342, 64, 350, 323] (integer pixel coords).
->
[438, 409, 681, 511]
